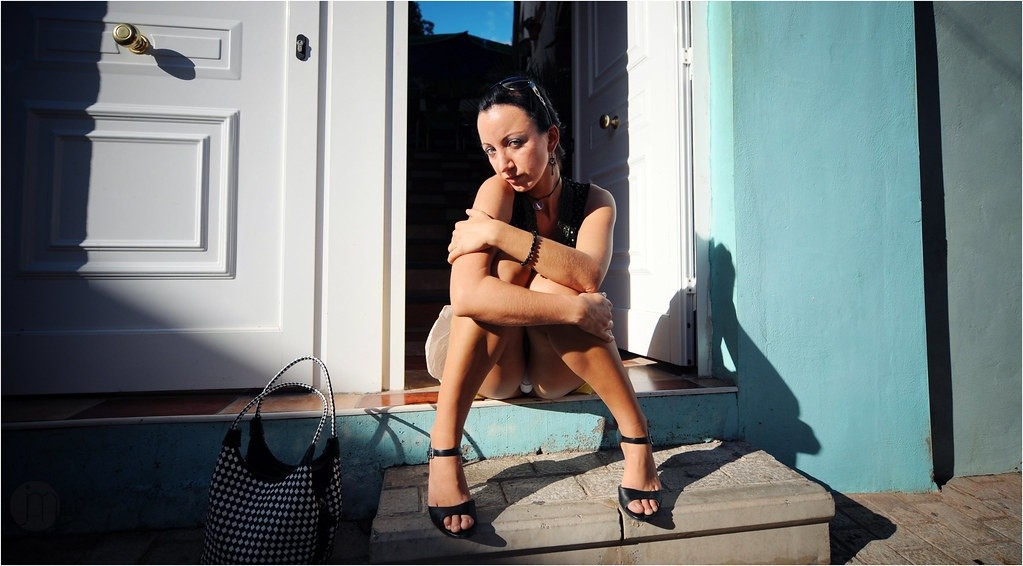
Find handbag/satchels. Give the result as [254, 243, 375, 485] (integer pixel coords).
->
[201, 357, 343, 565]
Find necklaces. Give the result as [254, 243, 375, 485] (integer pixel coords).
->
[524, 175, 561, 212]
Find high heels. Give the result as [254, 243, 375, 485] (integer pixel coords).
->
[427, 441, 476, 539]
[616, 426, 663, 521]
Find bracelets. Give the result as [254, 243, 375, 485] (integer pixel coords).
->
[520, 229, 539, 268]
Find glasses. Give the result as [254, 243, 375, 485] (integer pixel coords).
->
[484, 75, 552, 128]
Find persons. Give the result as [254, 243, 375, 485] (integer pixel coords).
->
[425, 77, 662, 538]
